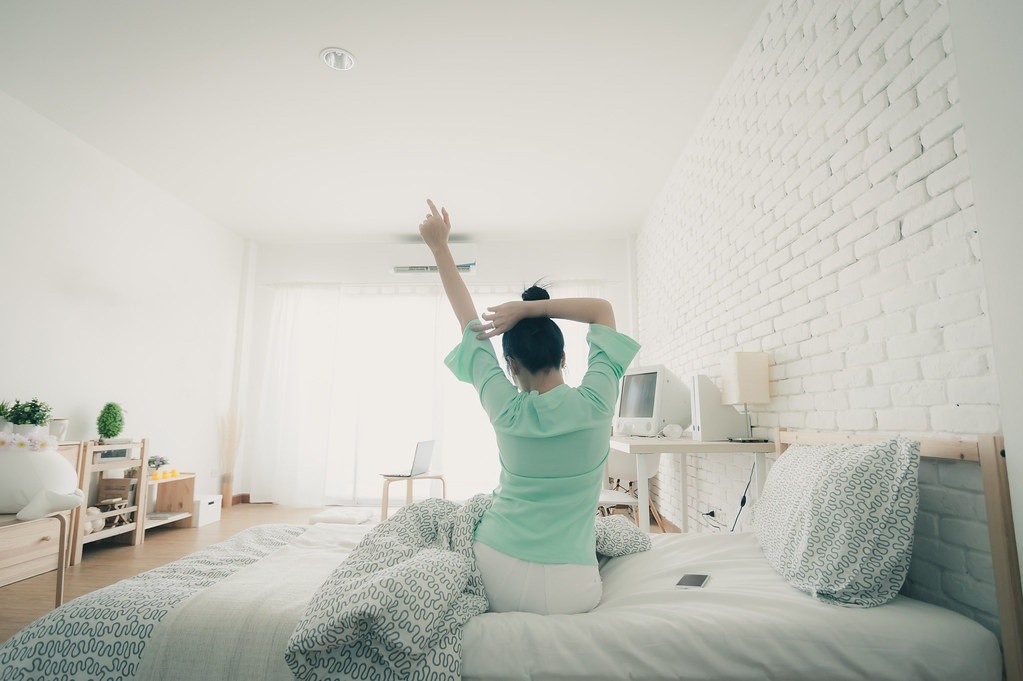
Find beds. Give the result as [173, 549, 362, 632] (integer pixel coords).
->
[0, 430, 1022, 681]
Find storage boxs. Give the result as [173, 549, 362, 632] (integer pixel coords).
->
[193, 494, 222, 529]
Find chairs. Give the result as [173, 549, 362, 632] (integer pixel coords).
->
[598, 453, 639, 527]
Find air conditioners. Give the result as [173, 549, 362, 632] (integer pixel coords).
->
[391, 241, 478, 271]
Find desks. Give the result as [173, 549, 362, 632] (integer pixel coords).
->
[609, 441, 777, 535]
[0, 440, 86, 566]
[382, 475, 447, 523]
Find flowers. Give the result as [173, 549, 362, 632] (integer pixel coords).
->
[9, 397, 53, 429]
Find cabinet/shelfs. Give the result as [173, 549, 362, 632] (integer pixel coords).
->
[71, 437, 150, 567]
[144, 473, 196, 536]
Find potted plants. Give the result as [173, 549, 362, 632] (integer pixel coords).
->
[97, 402, 133, 456]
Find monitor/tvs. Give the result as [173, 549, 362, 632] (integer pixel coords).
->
[613, 365, 692, 436]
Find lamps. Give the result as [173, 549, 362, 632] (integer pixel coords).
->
[722, 349, 774, 443]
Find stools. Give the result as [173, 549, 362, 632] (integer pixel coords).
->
[0, 510, 77, 610]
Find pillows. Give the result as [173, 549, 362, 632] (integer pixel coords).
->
[755, 439, 920, 608]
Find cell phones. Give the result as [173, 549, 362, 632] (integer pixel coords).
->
[676, 573, 710, 589]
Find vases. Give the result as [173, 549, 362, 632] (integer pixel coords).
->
[7, 421, 50, 441]
